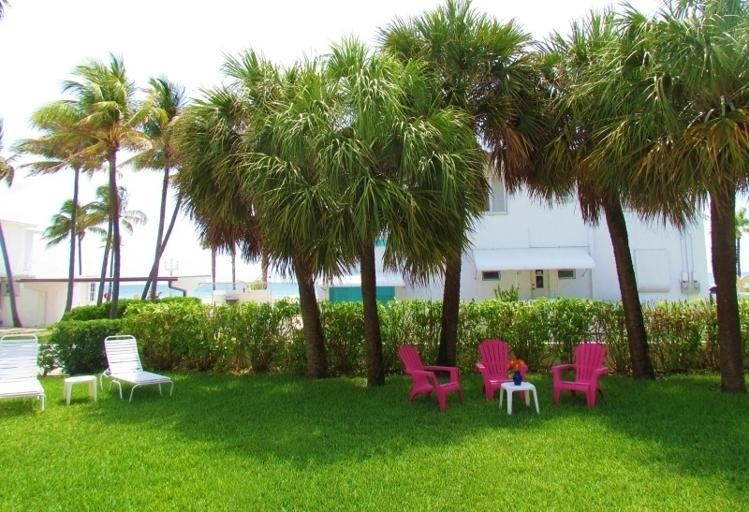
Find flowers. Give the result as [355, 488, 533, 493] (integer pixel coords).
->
[505, 356, 527, 373]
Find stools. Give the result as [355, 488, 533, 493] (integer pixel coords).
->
[64, 374, 98, 406]
[495, 379, 539, 419]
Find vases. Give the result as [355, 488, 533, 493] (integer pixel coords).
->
[513, 371, 521, 385]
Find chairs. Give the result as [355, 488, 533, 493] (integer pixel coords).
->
[400, 345, 462, 412]
[550, 342, 607, 406]
[0, 332, 47, 412]
[475, 337, 528, 401]
[99, 333, 175, 401]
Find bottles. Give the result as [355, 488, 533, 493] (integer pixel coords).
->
[513, 370, 522, 385]
